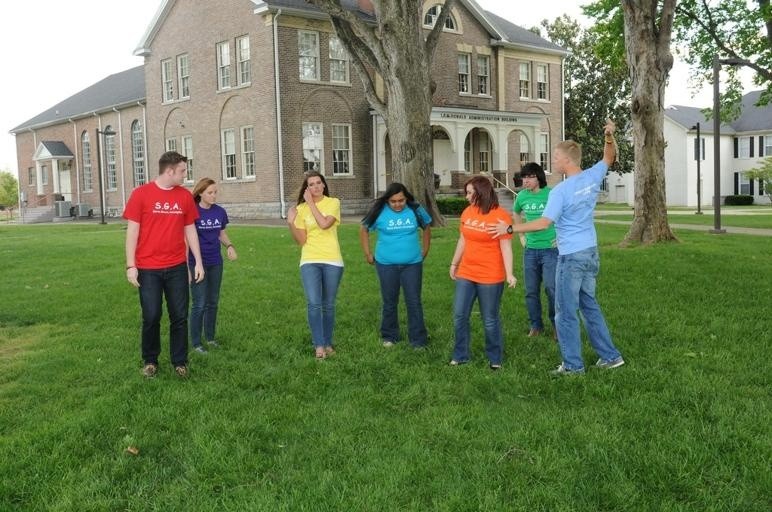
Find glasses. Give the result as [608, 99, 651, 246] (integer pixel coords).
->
[524, 174, 535, 178]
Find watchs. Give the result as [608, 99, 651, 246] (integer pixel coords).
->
[506, 224, 513, 234]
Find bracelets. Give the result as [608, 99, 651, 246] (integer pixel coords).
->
[605, 135, 616, 145]
[126, 266, 136, 270]
[450, 263, 459, 267]
[227, 243, 235, 250]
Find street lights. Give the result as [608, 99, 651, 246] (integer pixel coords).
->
[96, 126, 117, 225]
[689, 123, 703, 215]
[707, 52, 755, 235]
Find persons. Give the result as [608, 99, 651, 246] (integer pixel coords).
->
[359, 182, 433, 349]
[285, 169, 346, 361]
[512, 162, 558, 342]
[486, 115, 625, 381]
[183, 176, 238, 355]
[120, 150, 205, 381]
[447, 175, 518, 369]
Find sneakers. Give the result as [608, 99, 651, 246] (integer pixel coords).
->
[142, 341, 219, 379]
[315, 341, 424, 360]
[448, 327, 625, 377]
